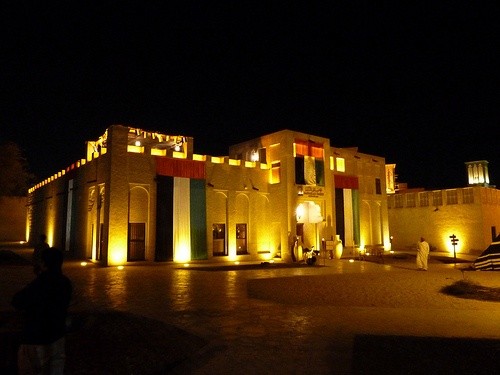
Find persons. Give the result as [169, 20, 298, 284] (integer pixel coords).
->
[415, 237, 430, 270]
[18, 247, 74, 375]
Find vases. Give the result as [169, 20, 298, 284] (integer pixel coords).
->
[293, 236, 304, 262]
[333, 235, 343, 259]
